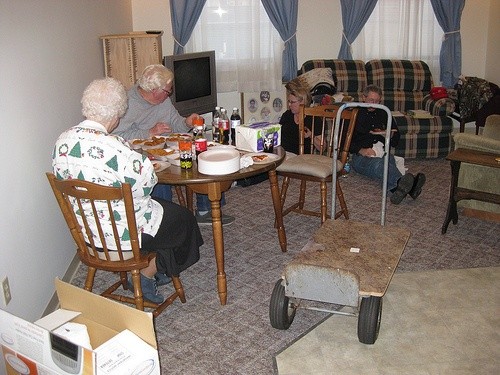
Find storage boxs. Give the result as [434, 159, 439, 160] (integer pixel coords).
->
[0, 277, 161, 375]
[235, 123, 281, 153]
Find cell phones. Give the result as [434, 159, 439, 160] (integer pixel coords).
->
[374, 129, 381, 132]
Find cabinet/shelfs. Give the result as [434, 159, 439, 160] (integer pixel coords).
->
[99, 30, 164, 91]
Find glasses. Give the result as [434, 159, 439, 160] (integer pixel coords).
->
[162, 87, 173, 98]
[287, 99, 302, 104]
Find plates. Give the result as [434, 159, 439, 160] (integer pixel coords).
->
[126, 132, 280, 175]
[369, 130, 385, 134]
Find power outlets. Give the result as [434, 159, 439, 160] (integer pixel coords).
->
[1, 277, 11, 306]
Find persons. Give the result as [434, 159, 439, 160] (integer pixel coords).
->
[339, 86, 426, 205]
[110, 65, 234, 226]
[232, 86, 327, 187]
[52, 78, 203, 304]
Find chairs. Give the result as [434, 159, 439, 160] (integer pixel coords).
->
[46, 172, 186, 319]
[274, 103, 359, 229]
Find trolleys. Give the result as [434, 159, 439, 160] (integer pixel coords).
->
[269, 102, 410, 345]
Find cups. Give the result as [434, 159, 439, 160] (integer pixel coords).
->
[178, 140, 192, 169]
[191, 116, 203, 126]
[263, 137, 273, 153]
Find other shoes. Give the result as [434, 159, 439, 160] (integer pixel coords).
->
[390, 172, 426, 205]
[194, 208, 236, 227]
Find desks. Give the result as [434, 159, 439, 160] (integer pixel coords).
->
[154, 133, 286, 306]
[442, 148, 500, 234]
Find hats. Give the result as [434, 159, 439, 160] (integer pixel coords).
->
[430, 87, 448, 99]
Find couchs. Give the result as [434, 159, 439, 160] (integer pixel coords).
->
[453, 115, 500, 223]
[297, 59, 454, 160]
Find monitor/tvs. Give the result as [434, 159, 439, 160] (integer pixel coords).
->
[161, 50, 218, 117]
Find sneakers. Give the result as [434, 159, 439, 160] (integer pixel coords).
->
[126, 271, 172, 304]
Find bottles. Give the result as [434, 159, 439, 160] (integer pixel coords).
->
[195, 126, 207, 165]
[211, 107, 222, 143]
[230, 108, 243, 146]
[218, 108, 230, 145]
